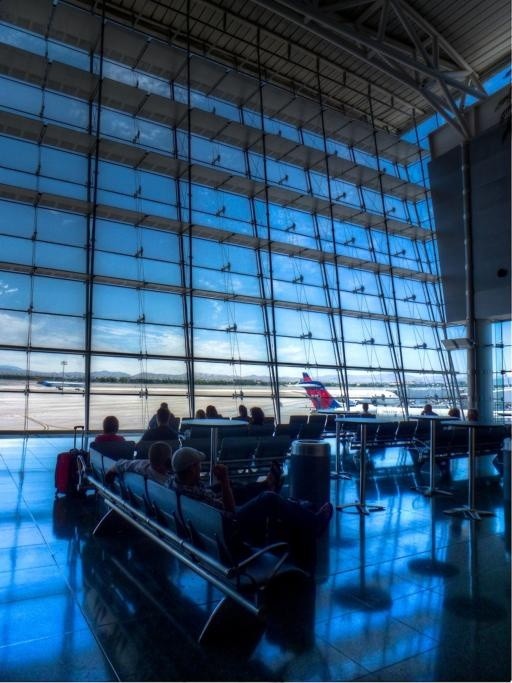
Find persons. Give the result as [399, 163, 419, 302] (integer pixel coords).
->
[246, 406, 276, 436]
[419, 403, 438, 417]
[147, 402, 179, 431]
[139, 407, 180, 441]
[467, 408, 479, 421]
[448, 407, 461, 420]
[104, 438, 287, 498]
[195, 408, 207, 419]
[165, 445, 334, 536]
[92, 415, 133, 459]
[236, 404, 251, 418]
[359, 402, 372, 417]
[204, 404, 223, 418]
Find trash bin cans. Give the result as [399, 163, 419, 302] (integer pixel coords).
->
[288, 439, 331, 503]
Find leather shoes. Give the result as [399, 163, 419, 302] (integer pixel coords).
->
[271, 460, 280, 479]
[317, 502, 332, 521]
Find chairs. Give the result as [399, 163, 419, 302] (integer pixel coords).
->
[77, 414, 512, 646]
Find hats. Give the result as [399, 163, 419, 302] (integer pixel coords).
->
[171, 446, 206, 472]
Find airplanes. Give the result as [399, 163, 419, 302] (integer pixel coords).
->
[285, 371, 511, 419]
[37, 379, 85, 390]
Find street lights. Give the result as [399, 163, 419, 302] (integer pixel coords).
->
[61, 360, 68, 394]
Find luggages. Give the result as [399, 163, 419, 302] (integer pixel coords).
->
[54, 425, 88, 496]
